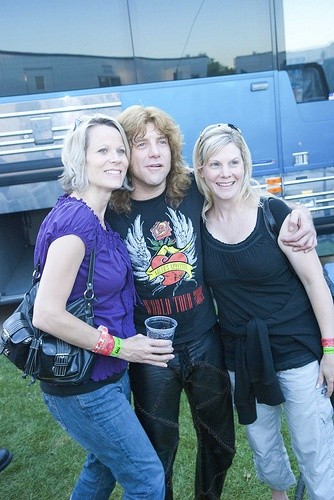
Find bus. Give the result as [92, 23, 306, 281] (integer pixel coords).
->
[0, 0, 334, 305]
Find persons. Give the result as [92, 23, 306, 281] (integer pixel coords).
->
[0, 448, 13, 473]
[31, 113, 175, 500]
[104, 104, 319, 500]
[192, 122, 334, 500]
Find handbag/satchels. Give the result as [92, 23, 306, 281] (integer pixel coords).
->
[323, 264, 334, 301]
[0, 247, 98, 387]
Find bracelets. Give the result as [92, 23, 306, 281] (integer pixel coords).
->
[323, 347, 334, 354]
[92, 324, 108, 354]
[100, 333, 115, 357]
[321, 338, 334, 347]
[110, 336, 123, 357]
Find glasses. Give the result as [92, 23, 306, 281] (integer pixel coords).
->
[198, 123, 242, 142]
[73, 113, 97, 132]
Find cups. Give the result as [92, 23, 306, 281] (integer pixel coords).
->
[144, 316, 178, 363]
